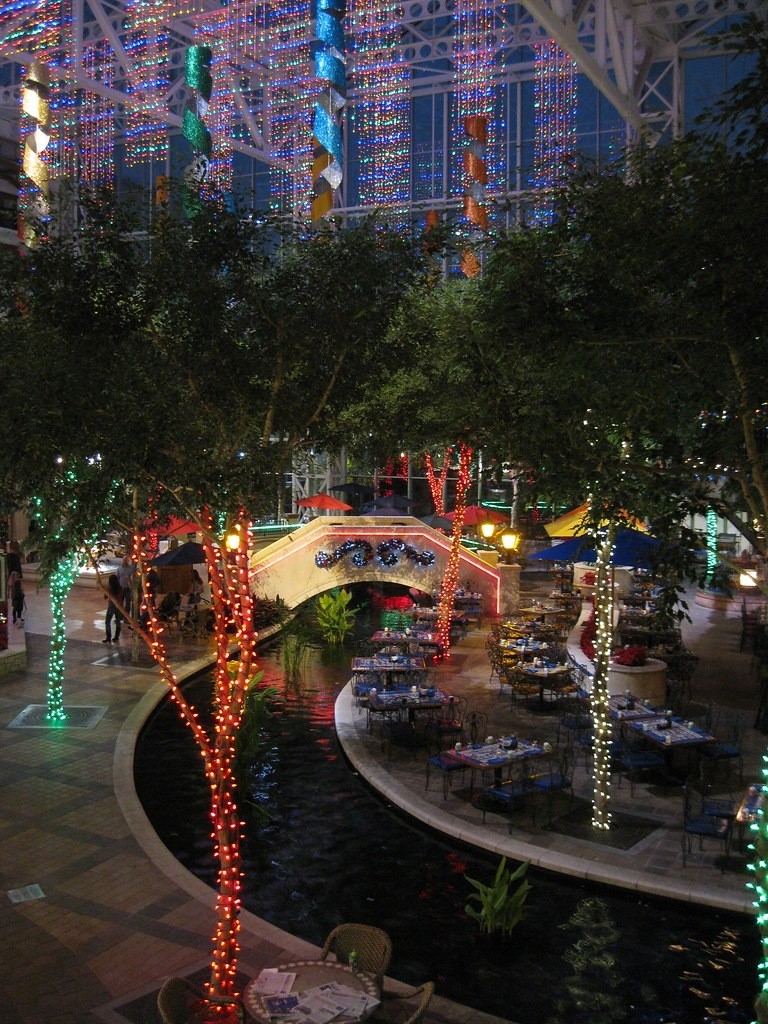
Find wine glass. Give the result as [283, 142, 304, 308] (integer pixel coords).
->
[532, 740, 538, 748]
[467, 743, 472, 750]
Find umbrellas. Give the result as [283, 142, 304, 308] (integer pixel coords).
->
[292, 494, 354, 516]
[359, 493, 693, 571]
[147, 541, 225, 568]
[328, 482, 378, 516]
[144, 512, 203, 537]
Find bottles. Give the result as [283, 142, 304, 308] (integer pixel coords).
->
[349, 948, 358, 973]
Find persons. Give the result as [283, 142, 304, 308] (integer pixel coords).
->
[139, 569, 204, 626]
[113, 555, 131, 624]
[101, 575, 123, 642]
[6, 541, 24, 623]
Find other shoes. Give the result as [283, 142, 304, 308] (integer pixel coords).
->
[17, 616, 24, 622]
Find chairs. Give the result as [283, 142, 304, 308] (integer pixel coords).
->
[367, 980, 435, 1024]
[157, 977, 247, 1024]
[178, 607, 210, 639]
[349, 598, 744, 874]
[317, 923, 393, 991]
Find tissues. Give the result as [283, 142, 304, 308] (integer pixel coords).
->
[499, 740, 518, 750]
[616, 702, 634, 709]
[657, 719, 671, 730]
[419, 689, 434, 698]
[535, 661, 548, 668]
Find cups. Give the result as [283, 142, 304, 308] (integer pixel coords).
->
[411, 686, 416, 693]
[643, 724, 650, 731]
[544, 742, 551, 751]
[645, 700, 650, 706]
[500, 637, 547, 651]
[743, 808, 749, 818]
[667, 709, 672, 717]
[487, 735, 494, 744]
[518, 656, 569, 675]
[508, 750, 516, 759]
[688, 722, 694, 729]
[749, 786, 756, 797]
[533, 606, 536, 610]
[456, 742, 461, 751]
[666, 735, 671, 743]
[507, 620, 548, 631]
[626, 690, 630, 697]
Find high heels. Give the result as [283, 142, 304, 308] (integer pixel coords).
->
[112, 637, 119, 641]
[103, 638, 111, 644]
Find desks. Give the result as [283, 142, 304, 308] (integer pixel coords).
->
[243, 960, 380, 1024]
[179, 605, 200, 626]
[351, 607, 768, 836]
